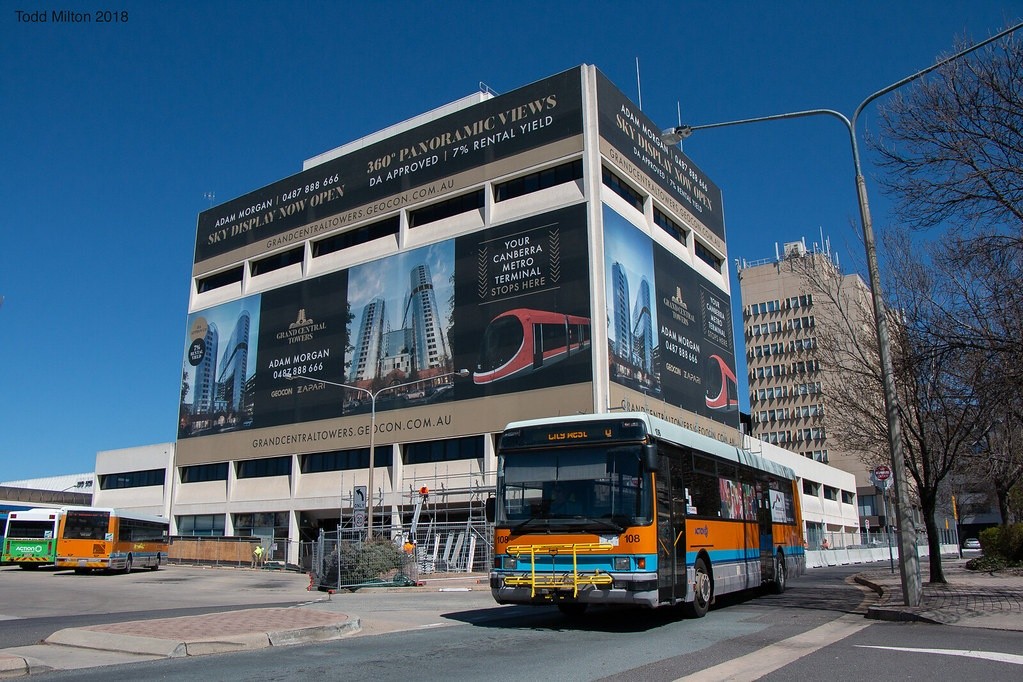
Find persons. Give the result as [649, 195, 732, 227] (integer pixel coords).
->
[823, 538, 830, 549]
[803, 539, 808, 548]
[419, 484, 429, 504]
[403, 539, 416, 555]
[251, 546, 265, 569]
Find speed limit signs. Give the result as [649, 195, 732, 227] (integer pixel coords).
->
[355, 513, 365, 527]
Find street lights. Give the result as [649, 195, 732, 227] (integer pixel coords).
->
[286, 369, 470, 539]
[658, 109, 921, 606]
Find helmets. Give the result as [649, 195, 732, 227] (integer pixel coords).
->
[412, 540, 418, 543]
[422, 484, 427, 487]
[262, 548, 265, 551]
[405, 539, 409, 542]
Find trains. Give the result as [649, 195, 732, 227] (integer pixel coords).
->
[704, 355, 738, 415]
[471, 308, 593, 388]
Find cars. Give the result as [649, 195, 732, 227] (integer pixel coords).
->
[402, 389, 425, 401]
[343, 400, 359, 409]
[392, 392, 405, 399]
[431, 383, 453, 395]
[243, 421, 252, 427]
[377, 396, 384, 400]
[964, 538, 980, 549]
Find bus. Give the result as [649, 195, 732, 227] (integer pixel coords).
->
[485, 410, 806, 621]
[55, 507, 174, 576]
[0, 505, 38, 555]
[1, 508, 90, 572]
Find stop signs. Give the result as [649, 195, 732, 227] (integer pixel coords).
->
[874, 466, 890, 481]
